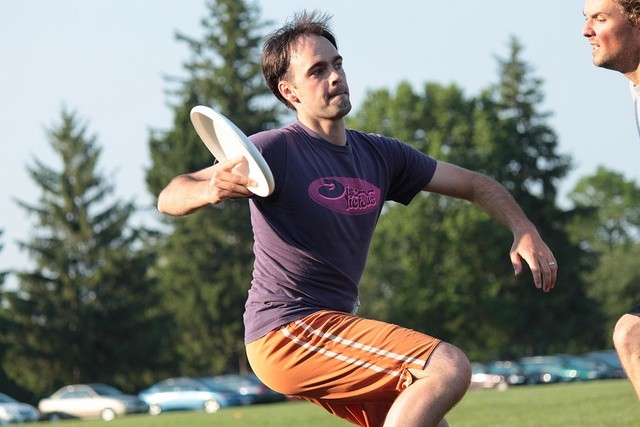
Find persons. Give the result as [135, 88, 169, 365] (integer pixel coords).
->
[578, 0, 640, 402]
[155, 5, 560, 427]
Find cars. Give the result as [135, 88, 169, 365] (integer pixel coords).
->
[469, 349, 628, 391]
[1, 393, 41, 426]
[38, 382, 149, 422]
[212, 374, 286, 406]
[137, 376, 241, 416]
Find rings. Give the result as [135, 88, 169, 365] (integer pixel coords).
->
[548, 260, 555, 266]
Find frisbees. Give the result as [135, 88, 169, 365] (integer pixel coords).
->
[190, 105, 275, 197]
